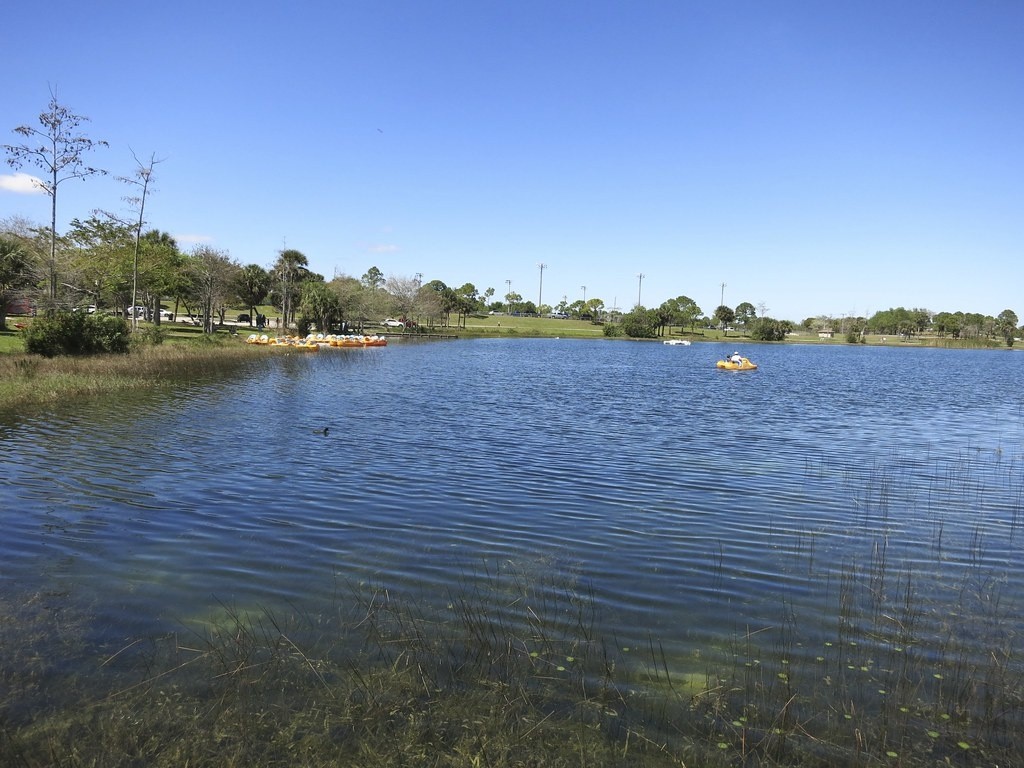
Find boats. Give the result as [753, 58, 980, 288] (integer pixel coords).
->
[717, 357, 759, 370]
[663, 339, 691, 346]
[246, 332, 389, 351]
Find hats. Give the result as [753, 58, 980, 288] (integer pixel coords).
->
[735, 351, 739, 355]
[727, 355, 730, 358]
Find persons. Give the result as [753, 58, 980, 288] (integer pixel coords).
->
[731, 351, 742, 367]
[256, 314, 262, 326]
[261, 314, 266, 325]
[276, 318, 280, 328]
[726, 355, 730, 362]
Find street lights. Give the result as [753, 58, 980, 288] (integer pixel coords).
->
[504, 278, 512, 310]
[719, 280, 727, 306]
[580, 285, 586, 301]
[636, 272, 646, 306]
[536, 263, 548, 308]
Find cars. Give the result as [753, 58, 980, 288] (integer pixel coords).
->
[72, 304, 95, 314]
[237, 314, 249, 323]
[128, 306, 173, 317]
[547, 311, 568, 320]
[398, 318, 417, 328]
[513, 308, 542, 318]
[380, 319, 403, 328]
